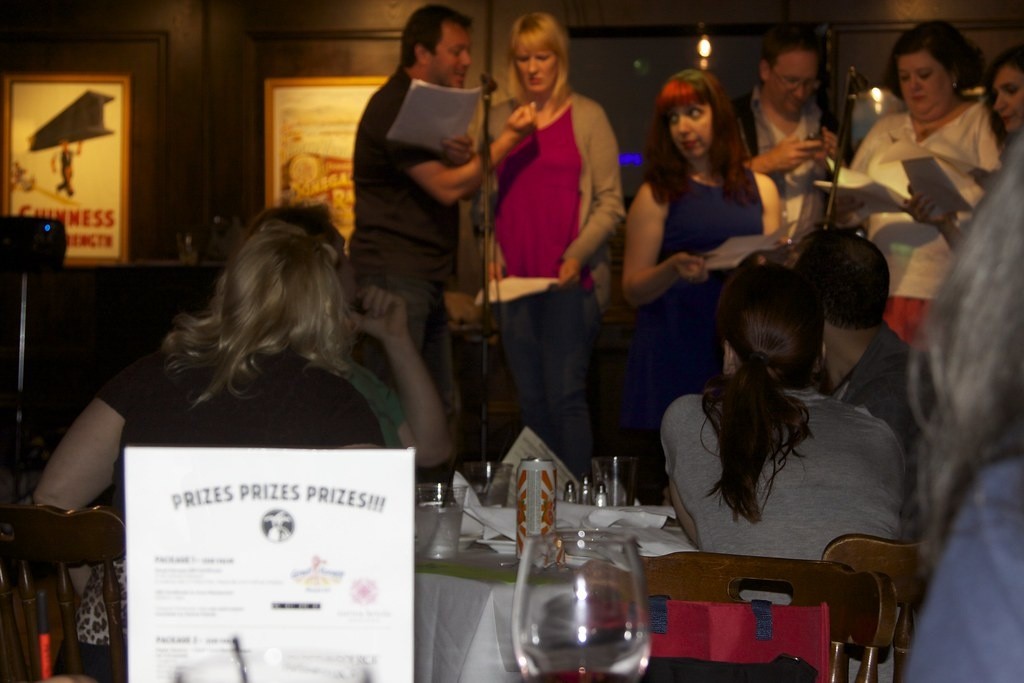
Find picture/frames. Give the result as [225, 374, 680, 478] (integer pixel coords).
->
[1, 74, 132, 268]
[264, 75, 390, 250]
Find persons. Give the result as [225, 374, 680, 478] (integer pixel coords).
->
[906, 125, 1024, 682]
[619, 18, 1024, 507]
[346, 5, 627, 487]
[33, 203, 458, 683]
[658, 227, 940, 607]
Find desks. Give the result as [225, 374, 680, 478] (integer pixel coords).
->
[417, 557, 650, 683]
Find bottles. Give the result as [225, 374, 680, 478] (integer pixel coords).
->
[562, 480, 577, 504]
[580, 475, 593, 506]
[593, 482, 609, 507]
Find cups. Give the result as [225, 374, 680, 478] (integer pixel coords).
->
[416, 483, 470, 562]
[510, 528, 651, 683]
[458, 460, 514, 509]
[591, 455, 636, 507]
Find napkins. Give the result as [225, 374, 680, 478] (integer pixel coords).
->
[454, 474, 677, 540]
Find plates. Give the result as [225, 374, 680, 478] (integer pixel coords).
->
[476, 537, 518, 554]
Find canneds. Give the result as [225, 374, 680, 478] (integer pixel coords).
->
[514, 457, 557, 560]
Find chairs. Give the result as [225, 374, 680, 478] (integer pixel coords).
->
[824, 535, 937, 683]
[579, 551, 897, 683]
[0, 502, 125, 683]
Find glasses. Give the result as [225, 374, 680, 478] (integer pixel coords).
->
[770, 65, 820, 90]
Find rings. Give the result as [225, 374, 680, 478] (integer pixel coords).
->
[688, 277, 693, 283]
